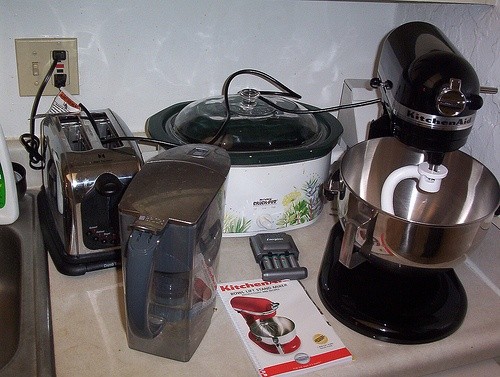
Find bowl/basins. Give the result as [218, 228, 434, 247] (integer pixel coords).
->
[249, 316, 296, 345]
[330, 137, 499, 271]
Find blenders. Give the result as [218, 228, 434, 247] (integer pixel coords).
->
[317, 21, 500, 346]
[231, 292, 301, 357]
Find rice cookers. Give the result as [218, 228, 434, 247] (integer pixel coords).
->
[147, 85, 345, 239]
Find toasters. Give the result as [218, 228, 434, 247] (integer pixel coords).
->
[38, 108, 151, 275]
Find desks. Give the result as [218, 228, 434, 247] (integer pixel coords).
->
[47, 194, 500, 377]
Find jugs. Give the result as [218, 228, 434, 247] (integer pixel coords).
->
[116, 142, 230, 359]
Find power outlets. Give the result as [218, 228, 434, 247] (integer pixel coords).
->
[15, 37, 79, 96]
